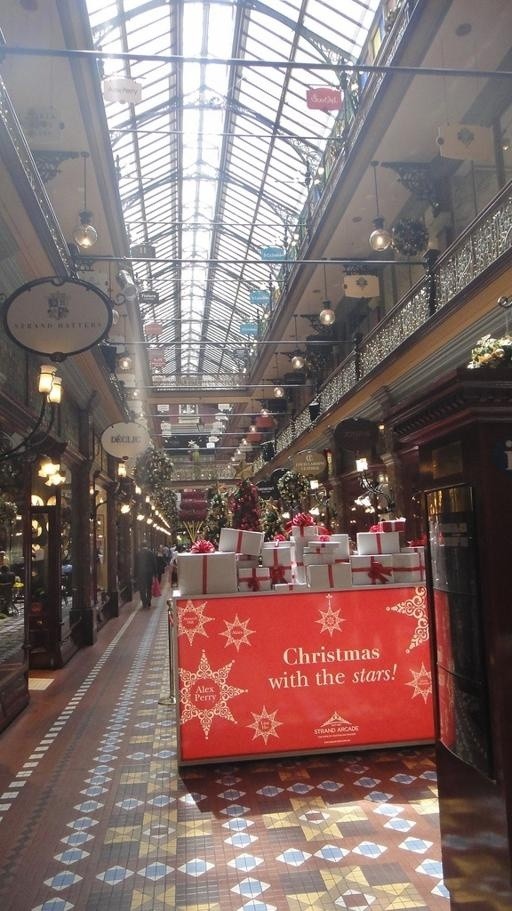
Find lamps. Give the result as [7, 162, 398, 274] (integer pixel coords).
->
[259, 158, 391, 419]
[1, 363, 68, 488]
[72, 151, 101, 251]
[116, 268, 137, 374]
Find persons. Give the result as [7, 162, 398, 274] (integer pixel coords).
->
[134, 539, 159, 608]
[63, 560, 72, 573]
[1, 565, 15, 585]
[151, 542, 189, 586]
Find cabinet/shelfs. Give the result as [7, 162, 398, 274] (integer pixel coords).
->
[416, 421, 510, 785]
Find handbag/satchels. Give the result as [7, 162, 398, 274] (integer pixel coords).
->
[152, 577, 160, 597]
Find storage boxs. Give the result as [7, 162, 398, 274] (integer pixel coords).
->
[175, 525, 427, 596]
